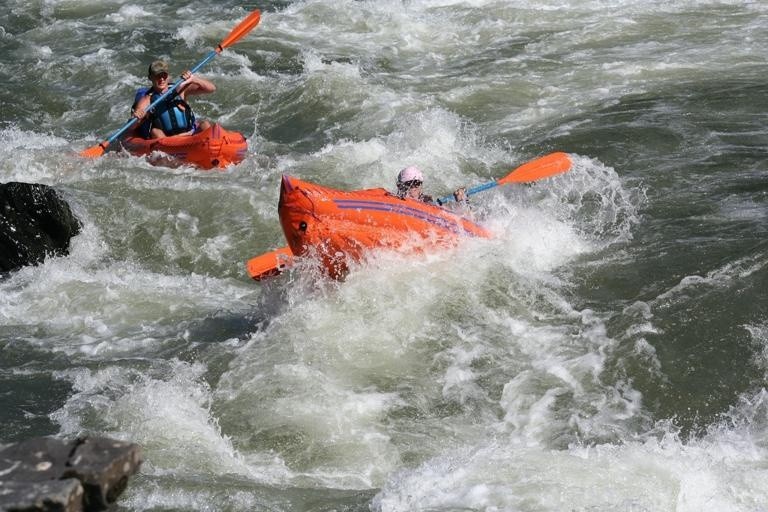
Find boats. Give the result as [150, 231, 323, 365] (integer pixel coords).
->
[278, 175, 491, 281]
[117, 118, 248, 170]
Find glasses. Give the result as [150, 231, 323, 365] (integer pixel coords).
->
[404, 182, 422, 190]
[154, 73, 168, 80]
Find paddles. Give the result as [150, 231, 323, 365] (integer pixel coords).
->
[82, 10, 261, 159]
[249, 150, 571, 282]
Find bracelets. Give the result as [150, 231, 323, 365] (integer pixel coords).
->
[460, 198, 470, 207]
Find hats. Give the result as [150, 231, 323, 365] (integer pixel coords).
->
[149, 60, 168, 77]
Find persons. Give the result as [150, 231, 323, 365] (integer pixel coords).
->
[127, 60, 216, 139]
[396, 166, 474, 217]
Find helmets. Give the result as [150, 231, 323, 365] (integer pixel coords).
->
[398, 166, 423, 186]
